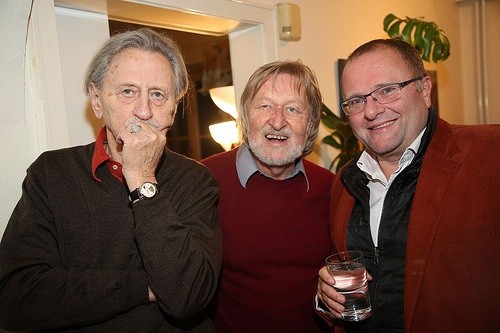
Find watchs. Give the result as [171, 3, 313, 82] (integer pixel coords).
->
[129, 181, 160, 203]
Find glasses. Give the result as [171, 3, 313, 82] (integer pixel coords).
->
[340, 75, 423, 117]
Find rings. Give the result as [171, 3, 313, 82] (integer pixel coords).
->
[130, 124, 143, 134]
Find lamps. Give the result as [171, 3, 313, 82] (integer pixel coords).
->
[277, 2, 303, 43]
[206, 84, 244, 153]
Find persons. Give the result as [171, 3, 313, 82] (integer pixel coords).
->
[0, 26, 221, 333]
[311, 38, 499, 333]
[196, 59, 339, 333]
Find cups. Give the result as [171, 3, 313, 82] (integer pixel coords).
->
[324, 249, 373, 322]
